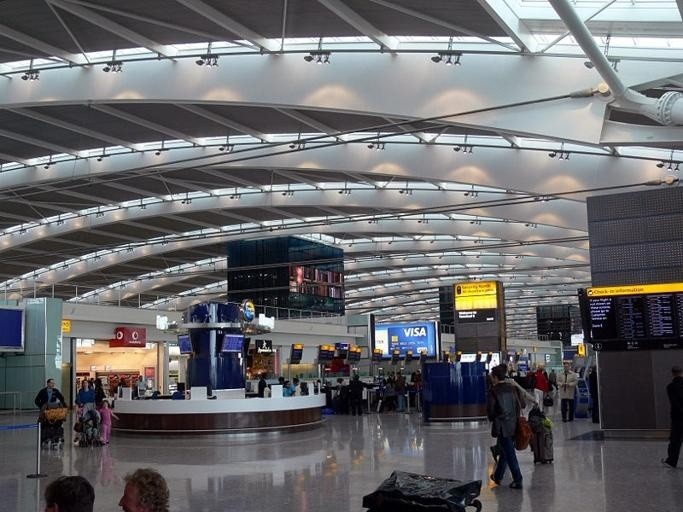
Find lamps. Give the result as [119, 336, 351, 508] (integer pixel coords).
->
[102, 48, 124, 73]
[583, 34, 622, 73]
[195, 40, 220, 66]
[432, 35, 464, 66]
[304, 37, 334, 65]
[21, 58, 40, 80]
[656, 148, 682, 172]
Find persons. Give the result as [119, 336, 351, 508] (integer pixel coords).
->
[45, 475, 95, 512]
[483, 363, 599, 490]
[119, 467, 170, 512]
[75, 376, 147, 447]
[660, 365, 683, 469]
[35, 379, 67, 410]
[256, 370, 422, 417]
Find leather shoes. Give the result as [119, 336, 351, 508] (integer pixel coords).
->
[491, 474, 500, 485]
[509, 481, 523, 490]
[490, 446, 498, 463]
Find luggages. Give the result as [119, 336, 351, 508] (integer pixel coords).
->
[528, 406, 554, 465]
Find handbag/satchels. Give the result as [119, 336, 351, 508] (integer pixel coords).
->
[543, 395, 554, 407]
[515, 416, 533, 451]
[39, 401, 68, 441]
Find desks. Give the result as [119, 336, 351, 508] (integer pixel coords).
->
[0, 391, 22, 415]
